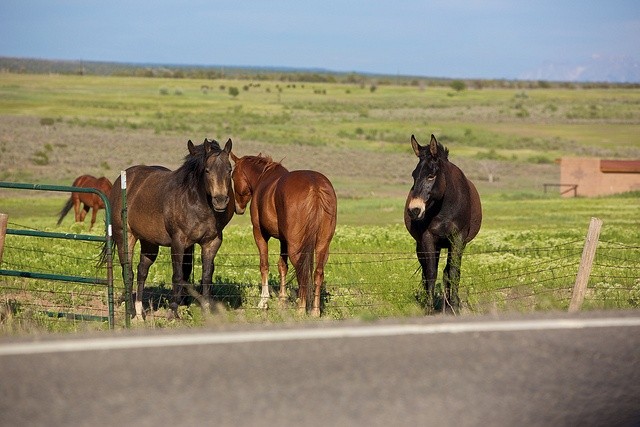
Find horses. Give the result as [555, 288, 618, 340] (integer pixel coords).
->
[56, 175, 112, 232]
[149, 138, 214, 305]
[93, 138, 236, 322]
[230, 151, 337, 317]
[404, 134, 482, 316]
